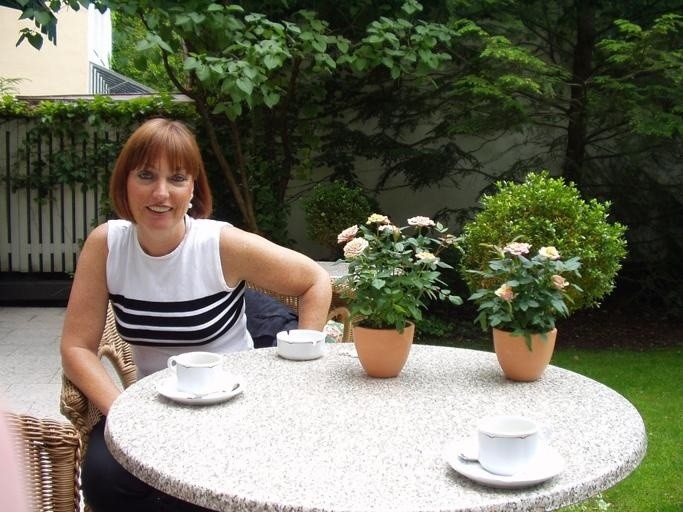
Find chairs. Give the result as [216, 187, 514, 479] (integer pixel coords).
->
[0, 412, 80, 512]
[60, 280, 370, 512]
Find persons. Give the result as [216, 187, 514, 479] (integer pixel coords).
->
[60, 118, 334, 510]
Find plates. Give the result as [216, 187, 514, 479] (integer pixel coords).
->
[156, 377, 245, 407]
[438, 438, 567, 488]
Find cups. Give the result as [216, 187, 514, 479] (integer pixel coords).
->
[473, 415, 538, 475]
[165, 350, 224, 395]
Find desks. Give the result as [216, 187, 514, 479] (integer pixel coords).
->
[103, 343, 649, 512]
[316, 262, 406, 282]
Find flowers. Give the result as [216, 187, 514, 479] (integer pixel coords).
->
[466, 235, 584, 352]
[334, 214, 464, 332]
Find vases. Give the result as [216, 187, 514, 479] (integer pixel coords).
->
[351, 320, 415, 377]
[492, 328, 557, 381]
